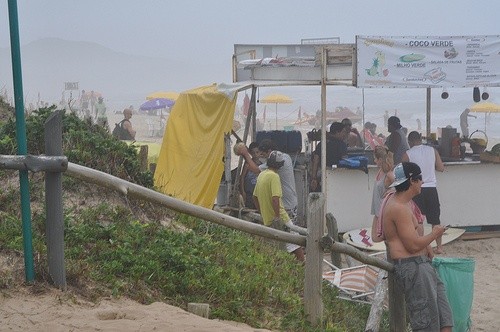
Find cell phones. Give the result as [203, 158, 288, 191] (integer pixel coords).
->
[444, 224, 450, 228]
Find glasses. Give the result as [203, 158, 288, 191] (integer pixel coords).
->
[344, 123, 351, 126]
[414, 175, 422, 181]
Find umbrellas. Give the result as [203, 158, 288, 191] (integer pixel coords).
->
[469, 101, 500, 133]
[140, 98, 175, 111]
[260, 92, 295, 130]
[86, 91, 102, 97]
[148, 92, 179, 119]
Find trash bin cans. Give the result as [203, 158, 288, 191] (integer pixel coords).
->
[431, 258, 475, 332]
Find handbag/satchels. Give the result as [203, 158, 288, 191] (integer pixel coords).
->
[307, 128, 318, 141]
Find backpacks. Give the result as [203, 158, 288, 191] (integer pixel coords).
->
[112, 119, 131, 140]
[339, 156, 369, 174]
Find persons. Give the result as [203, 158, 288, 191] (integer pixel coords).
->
[460, 111, 476, 137]
[121, 108, 136, 140]
[360, 122, 385, 152]
[240, 138, 298, 224]
[80, 90, 107, 125]
[377, 162, 454, 332]
[371, 166, 424, 242]
[384, 116, 409, 160]
[240, 142, 265, 209]
[251, 152, 304, 261]
[371, 147, 394, 215]
[401, 131, 444, 253]
[342, 118, 364, 147]
[310, 122, 345, 192]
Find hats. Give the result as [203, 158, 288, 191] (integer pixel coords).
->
[268, 150, 284, 161]
[389, 161, 421, 187]
